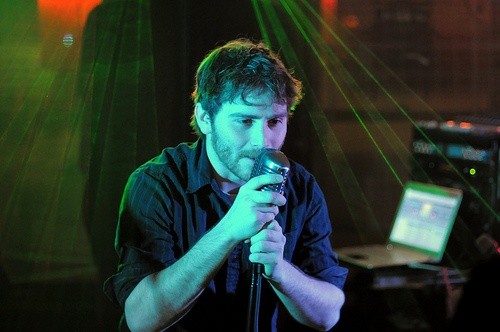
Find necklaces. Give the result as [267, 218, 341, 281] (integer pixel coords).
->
[220, 188, 238, 197]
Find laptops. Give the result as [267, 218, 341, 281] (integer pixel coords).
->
[333, 182, 463, 269]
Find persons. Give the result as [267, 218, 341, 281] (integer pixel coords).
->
[110, 38, 348, 331]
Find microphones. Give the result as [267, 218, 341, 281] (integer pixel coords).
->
[251, 148, 289, 208]
